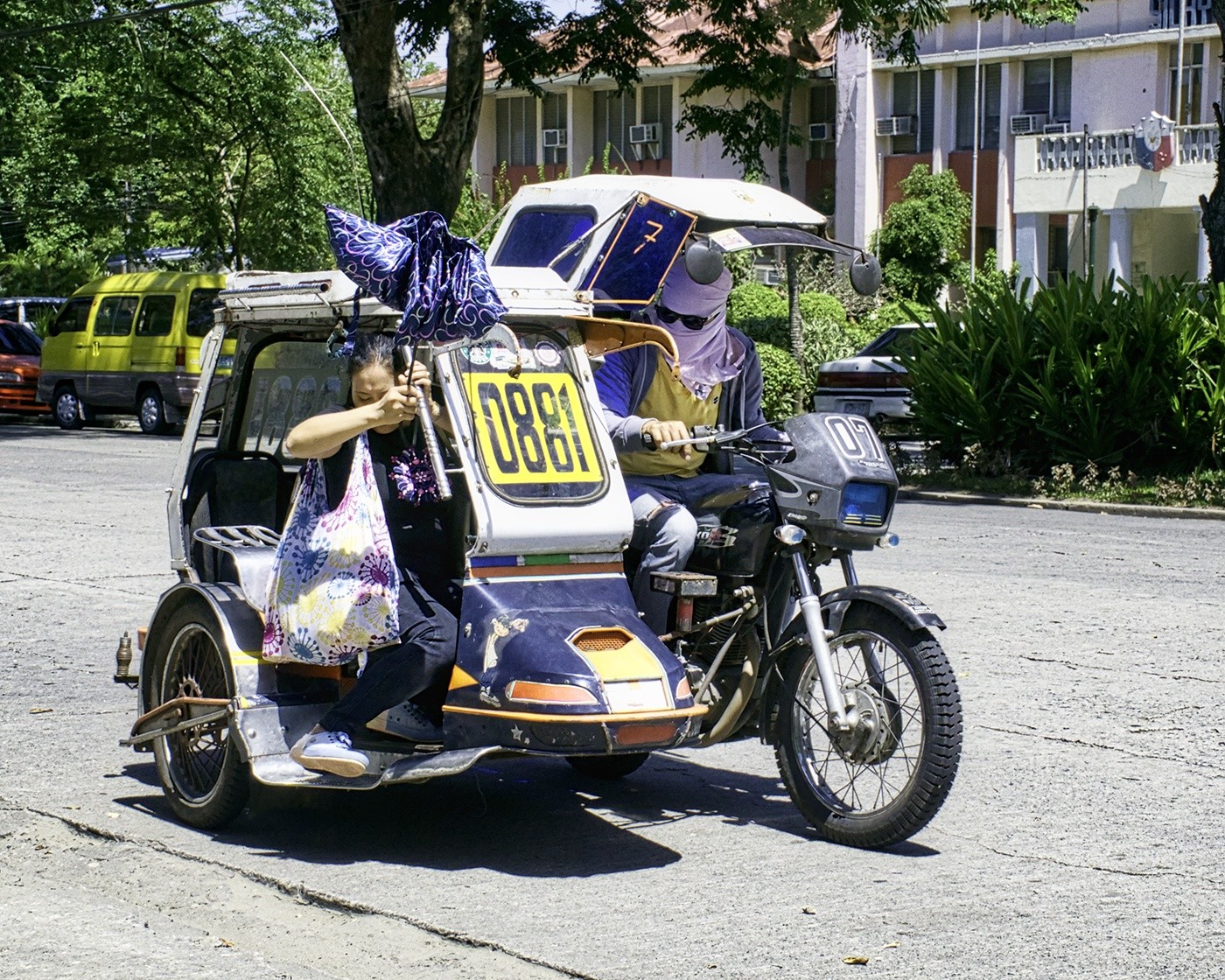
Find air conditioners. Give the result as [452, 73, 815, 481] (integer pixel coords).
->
[1044, 122, 1070, 136]
[629, 123, 661, 143]
[876, 116, 913, 136]
[543, 129, 568, 148]
[810, 123, 835, 141]
[1010, 113, 1048, 134]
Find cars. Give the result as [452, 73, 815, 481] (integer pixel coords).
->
[1, 320, 54, 418]
[812, 320, 962, 437]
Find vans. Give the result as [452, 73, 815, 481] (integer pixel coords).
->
[40, 271, 282, 435]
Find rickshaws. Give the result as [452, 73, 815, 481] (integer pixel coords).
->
[110, 168, 963, 855]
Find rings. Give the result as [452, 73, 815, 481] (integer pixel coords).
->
[427, 371, 430, 378]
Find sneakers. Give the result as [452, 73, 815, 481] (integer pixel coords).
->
[290, 729, 370, 778]
[366, 697, 443, 744]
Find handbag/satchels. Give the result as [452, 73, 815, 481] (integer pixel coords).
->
[261, 405, 403, 668]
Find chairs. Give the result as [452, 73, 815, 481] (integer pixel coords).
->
[187, 448, 285, 583]
[113, 310, 165, 336]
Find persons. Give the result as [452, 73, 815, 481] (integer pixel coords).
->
[591, 258, 783, 636]
[286, 334, 460, 776]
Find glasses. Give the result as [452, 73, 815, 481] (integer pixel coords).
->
[652, 288, 726, 330]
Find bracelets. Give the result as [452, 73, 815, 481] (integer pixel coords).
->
[434, 402, 442, 422]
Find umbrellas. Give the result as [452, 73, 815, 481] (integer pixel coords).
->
[322, 203, 510, 383]
